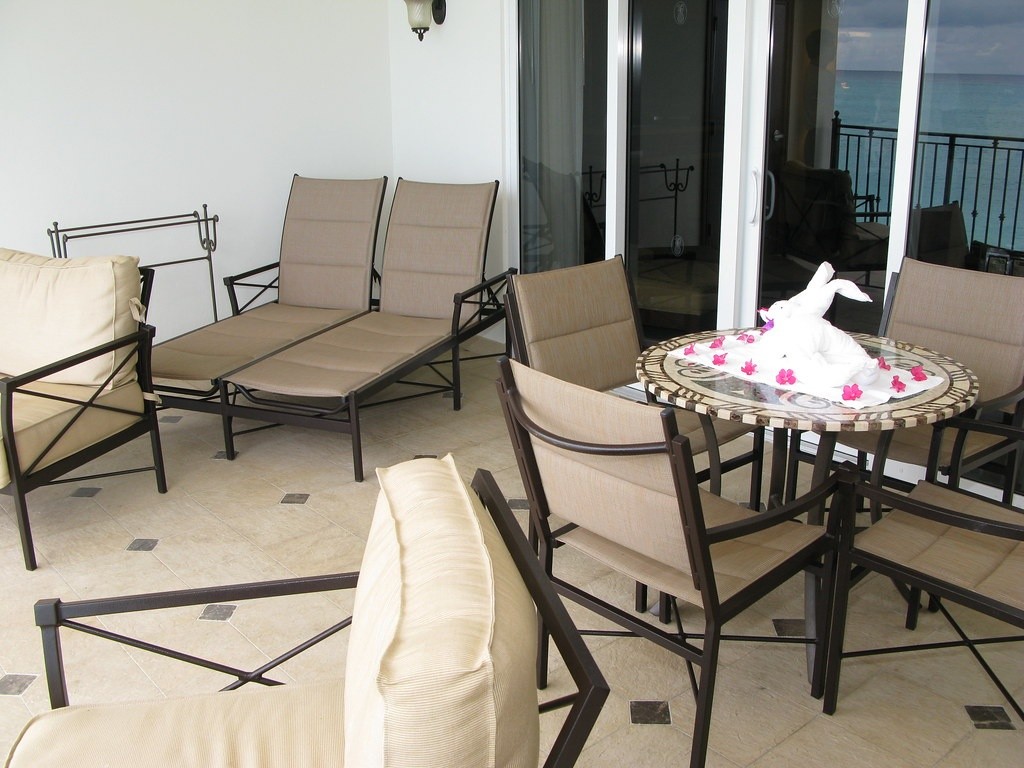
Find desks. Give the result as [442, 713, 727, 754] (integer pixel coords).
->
[633, 328, 981, 687]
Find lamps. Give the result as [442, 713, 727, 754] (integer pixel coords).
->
[404, 0, 446, 41]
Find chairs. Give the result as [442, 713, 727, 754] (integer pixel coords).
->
[217, 176, 508, 482]
[909, 201, 970, 271]
[152, 174, 389, 462]
[821, 415, 1024, 721]
[0, 248, 167, 571]
[0, 452, 611, 768]
[503, 253, 766, 625]
[785, 256, 1023, 613]
[777, 160, 892, 327]
[493, 355, 857, 767]
